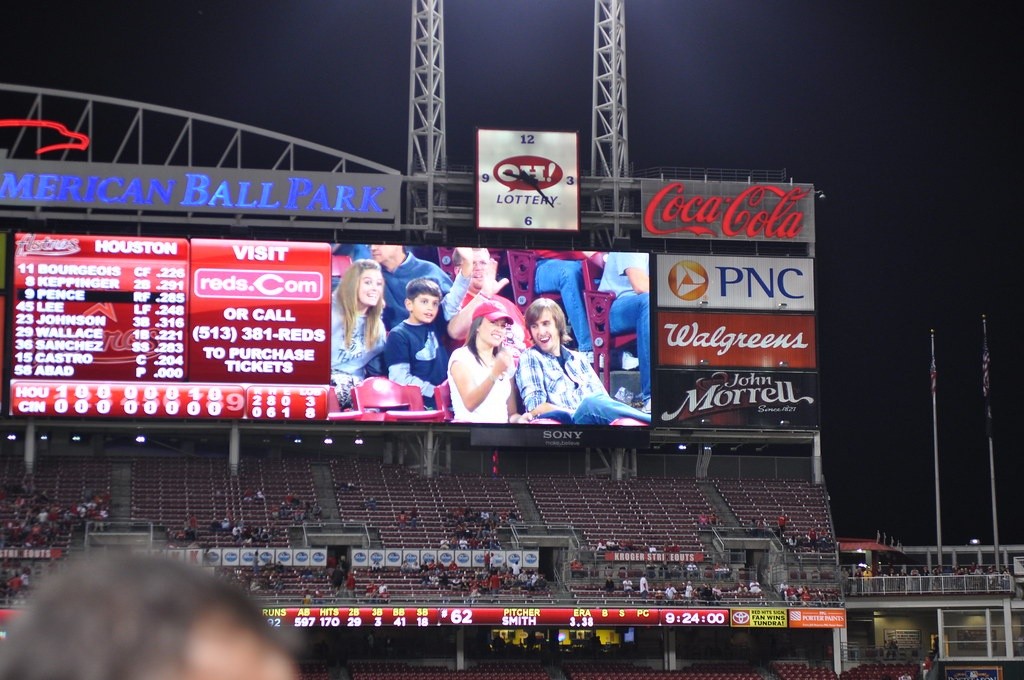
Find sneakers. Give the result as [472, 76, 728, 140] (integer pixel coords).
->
[599, 350, 639, 371]
[581, 350, 605, 369]
[642, 398, 651, 413]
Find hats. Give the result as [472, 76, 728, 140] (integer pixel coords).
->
[472, 301, 514, 325]
[688, 581, 692, 585]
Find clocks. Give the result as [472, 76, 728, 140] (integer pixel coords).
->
[472, 126, 582, 233]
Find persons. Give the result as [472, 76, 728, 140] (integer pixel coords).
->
[368, 497, 517, 550]
[0, 547, 301, 680]
[569, 511, 845, 607]
[164, 487, 323, 548]
[922, 656, 931, 680]
[0, 487, 112, 606]
[338, 480, 356, 493]
[216, 567, 357, 606]
[366, 552, 547, 604]
[1018, 633, 1024, 656]
[931, 636, 940, 658]
[886, 638, 898, 660]
[841, 565, 1012, 597]
[880, 672, 912, 680]
[331, 245, 651, 425]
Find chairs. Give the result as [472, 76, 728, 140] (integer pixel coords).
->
[0, 453, 925, 680]
